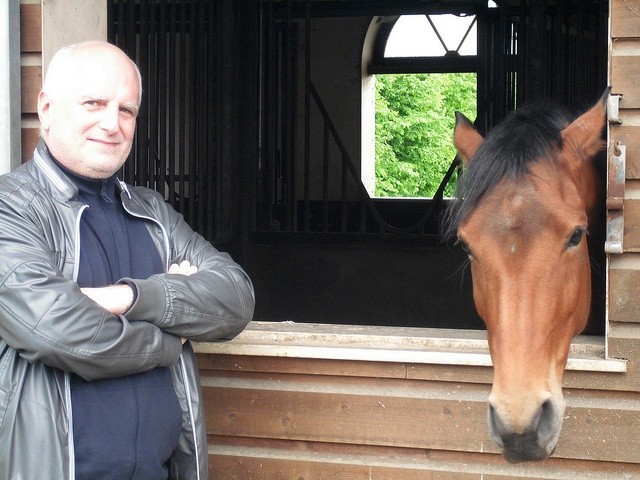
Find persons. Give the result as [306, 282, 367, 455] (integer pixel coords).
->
[0, 41, 256, 480]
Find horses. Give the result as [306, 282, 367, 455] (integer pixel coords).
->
[434, 85, 614, 465]
